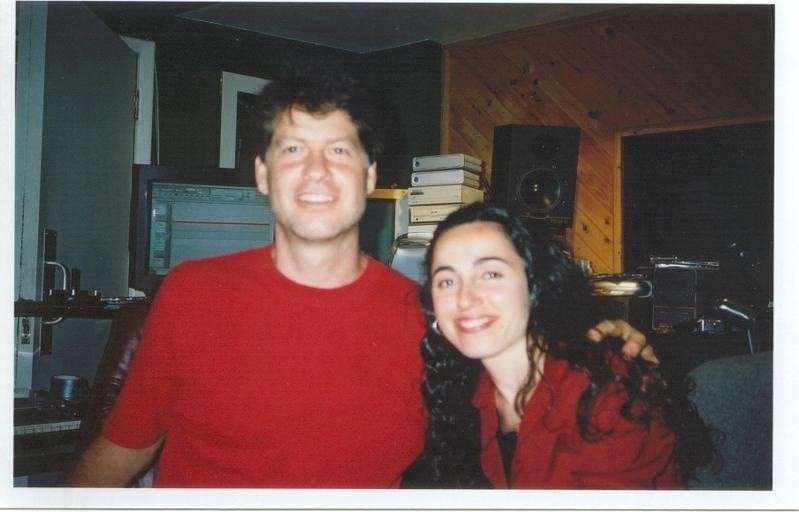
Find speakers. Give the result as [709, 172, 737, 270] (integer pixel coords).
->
[491, 122, 581, 234]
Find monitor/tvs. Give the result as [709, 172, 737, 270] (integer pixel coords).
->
[128, 162, 276, 300]
[358, 187, 411, 268]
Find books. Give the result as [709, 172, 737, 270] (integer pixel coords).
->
[411, 153, 483, 173]
[408, 186, 485, 205]
[406, 224, 438, 241]
[410, 171, 481, 188]
[407, 206, 474, 226]
[399, 235, 432, 249]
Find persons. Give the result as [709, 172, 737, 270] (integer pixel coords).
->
[57, 58, 662, 489]
[398, 198, 729, 490]
